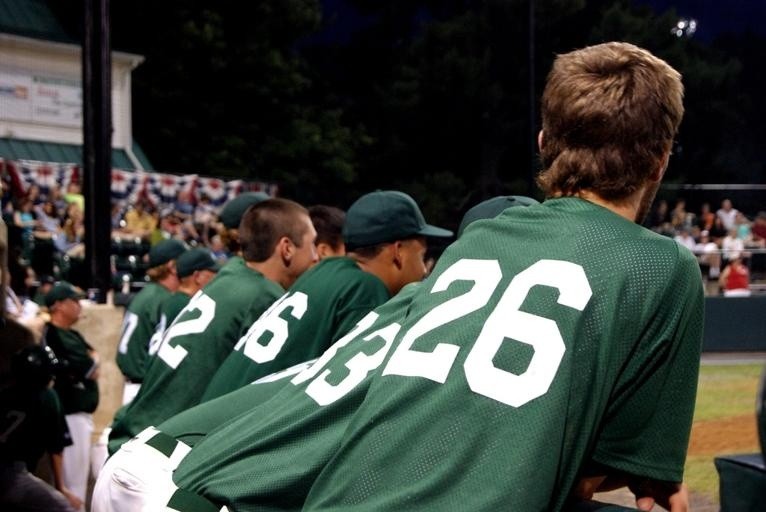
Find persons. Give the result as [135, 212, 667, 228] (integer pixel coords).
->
[0, 42, 765, 511]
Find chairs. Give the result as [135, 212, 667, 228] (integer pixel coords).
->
[0, 160, 280, 298]
[647, 185, 766, 296]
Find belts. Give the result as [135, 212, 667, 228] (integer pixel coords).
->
[147, 432, 178, 457]
[167, 489, 224, 512]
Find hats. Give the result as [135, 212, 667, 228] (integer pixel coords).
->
[457, 196, 542, 238]
[221, 191, 268, 228]
[46, 285, 85, 306]
[150, 239, 222, 278]
[343, 189, 453, 248]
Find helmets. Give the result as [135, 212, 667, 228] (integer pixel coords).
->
[13, 345, 58, 377]
